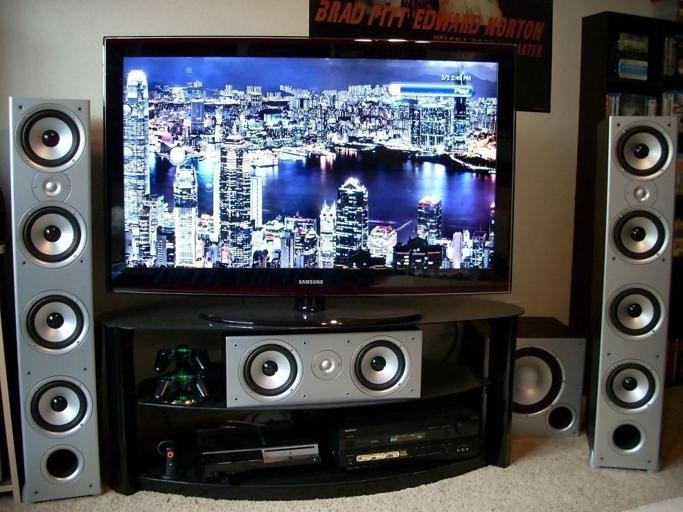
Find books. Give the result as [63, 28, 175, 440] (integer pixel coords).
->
[607, 30, 682, 260]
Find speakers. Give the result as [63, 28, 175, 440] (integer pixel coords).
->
[226, 326, 425, 408]
[7, 93, 106, 502]
[511, 317, 587, 439]
[569, 116, 678, 472]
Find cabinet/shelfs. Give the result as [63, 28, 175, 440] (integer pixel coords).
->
[567, 10, 683, 388]
[100, 293, 524, 504]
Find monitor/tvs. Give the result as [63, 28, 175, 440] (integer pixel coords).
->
[102, 33, 517, 321]
